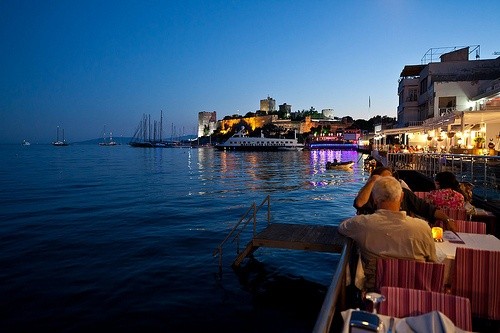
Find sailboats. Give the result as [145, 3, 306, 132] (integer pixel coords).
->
[51, 123, 71, 146]
[98, 125, 117, 146]
[128, 110, 198, 147]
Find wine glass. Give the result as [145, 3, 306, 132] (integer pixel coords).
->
[365, 293, 386, 314]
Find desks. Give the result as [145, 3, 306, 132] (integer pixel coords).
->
[434, 231, 500, 284]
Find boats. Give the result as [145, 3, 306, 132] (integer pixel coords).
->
[23, 140, 31, 146]
[364, 155, 376, 166]
[215, 126, 305, 151]
[278, 145, 304, 152]
[326, 160, 355, 170]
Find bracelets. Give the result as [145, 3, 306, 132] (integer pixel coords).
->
[444, 218, 455, 224]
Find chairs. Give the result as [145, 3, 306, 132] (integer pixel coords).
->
[375, 192, 500, 333]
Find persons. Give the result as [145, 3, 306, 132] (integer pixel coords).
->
[339, 176, 440, 292]
[426, 172, 490, 232]
[391, 143, 419, 153]
[353, 167, 457, 233]
